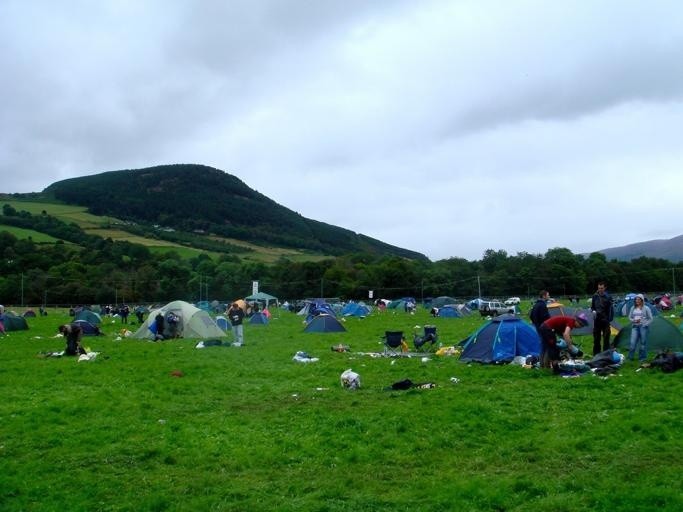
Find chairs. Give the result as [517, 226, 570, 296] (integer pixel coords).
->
[416, 327, 436, 353]
[384, 331, 405, 359]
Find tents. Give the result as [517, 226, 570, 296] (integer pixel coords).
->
[22, 309, 36, 318]
[0, 309, 30, 332]
[374, 295, 485, 319]
[69, 304, 103, 337]
[456, 312, 546, 364]
[545, 291, 682, 352]
[127, 298, 228, 342]
[197, 296, 370, 334]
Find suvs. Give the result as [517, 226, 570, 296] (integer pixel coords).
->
[479, 302, 514, 317]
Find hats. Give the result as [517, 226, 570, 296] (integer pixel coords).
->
[575, 314, 588, 326]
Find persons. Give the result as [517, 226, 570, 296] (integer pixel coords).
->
[539, 311, 589, 367]
[228, 303, 244, 348]
[0, 305, 6, 334]
[57, 323, 86, 355]
[626, 291, 653, 362]
[590, 282, 614, 356]
[167, 312, 180, 339]
[39, 305, 44, 316]
[529, 290, 551, 331]
[155, 311, 164, 341]
[103, 301, 144, 324]
